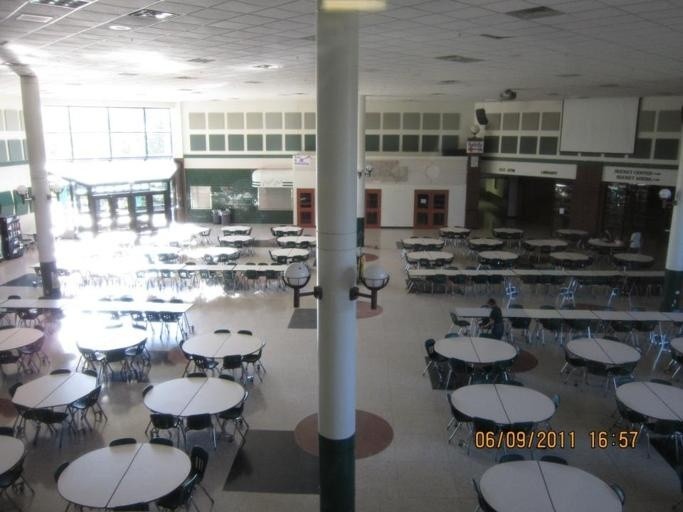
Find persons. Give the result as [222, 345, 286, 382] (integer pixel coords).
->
[480, 298, 505, 341]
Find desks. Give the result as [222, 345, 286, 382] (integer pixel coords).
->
[204, 246, 241, 263]
[556, 228, 590, 236]
[476, 458, 624, 512]
[524, 238, 568, 247]
[277, 235, 317, 245]
[548, 251, 591, 262]
[142, 376, 246, 417]
[530, 270, 660, 311]
[271, 226, 303, 233]
[614, 380, 683, 425]
[75, 329, 149, 353]
[0, 298, 62, 311]
[56, 441, 192, 509]
[10, 373, 97, 409]
[470, 238, 504, 246]
[613, 253, 656, 263]
[181, 333, 267, 360]
[439, 227, 470, 234]
[402, 237, 443, 247]
[0, 326, 44, 351]
[221, 224, 254, 233]
[479, 250, 519, 261]
[1, 435, 28, 477]
[587, 237, 626, 248]
[493, 227, 524, 234]
[406, 250, 454, 265]
[270, 247, 311, 259]
[219, 235, 255, 243]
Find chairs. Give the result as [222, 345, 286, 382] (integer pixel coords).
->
[241, 344, 266, 384]
[108, 437, 137, 447]
[218, 391, 250, 444]
[535, 395, 561, 432]
[214, 328, 231, 333]
[444, 393, 469, 444]
[75, 343, 104, 375]
[15, 306, 42, 328]
[193, 356, 219, 377]
[49, 368, 71, 375]
[187, 373, 206, 378]
[125, 336, 149, 385]
[185, 260, 286, 265]
[237, 329, 253, 336]
[501, 419, 535, 461]
[114, 502, 149, 511]
[218, 374, 235, 381]
[403, 271, 528, 311]
[490, 451, 525, 465]
[162, 267, 285, 296]
[96, 347, 129, 384]
[149, 413, 186, 449]
[30, 407, 69, 449]
[158, 226, 257, 260]
[150, 438, 173, 447]
[142, 384, 159, 439]
[16, 336, 50, 377]
[644, 417, 682, 463]
[221, 354, 244, 380]
[9, 382, 25, 430]
[155, 472, 201, 512]
[0, 422, 16, 437]
[0, 448, 35, 512]
[0, 348, 25, 385]
[268, 222, 317, 263]
[0, 324, 16, 330]
[102, 296, 186, 304]
[34, 324, 46, 332]
[82, 369, 97, 377]
[53, 462, 84, 512]
[80, 307, 194, 346]
[68, 386, 108, 433]
[178, 339, 194, 379]
[470, 475, 497, 512]
[420, 310, 683, 392]
[394, 223, 510, 273]
[464, 414, 502, 455]
[540, 451, 566, 463]
[606, 393, 646, 438]
[609, 479, 624, 507]
[183, 412, 214, 449]
[190, 444, 216, 505]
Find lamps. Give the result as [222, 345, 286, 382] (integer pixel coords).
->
[658, 189, 674, 208]
[17, 185, 36, 205]
[356, 166, 364, 179]
[364, 164, 373, 176]
[470, 124, 481, 137]
[47, 187, 63, 199]
